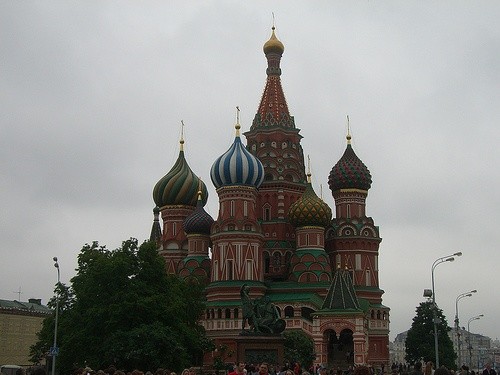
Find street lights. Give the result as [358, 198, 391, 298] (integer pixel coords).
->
[424, 252, 463, 370]
[467, 315, 484, 361]
[52, 257, 60, 375]
[454, 290, 478, 356]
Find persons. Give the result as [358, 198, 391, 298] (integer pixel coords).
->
[0, 350, 500, 375]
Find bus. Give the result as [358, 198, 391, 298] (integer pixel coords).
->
[0, 365, 24, 375]
